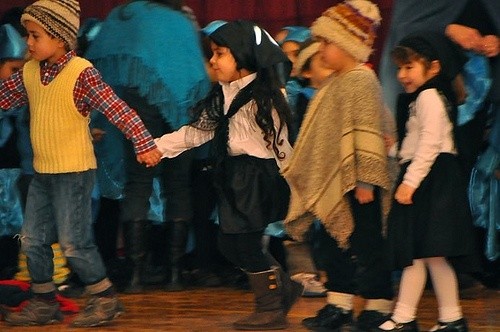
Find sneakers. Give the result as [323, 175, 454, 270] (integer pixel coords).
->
[70, 297, 126, 328]
[4, 301, 63, 326]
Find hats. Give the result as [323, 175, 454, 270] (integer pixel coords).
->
[311, 0, 382, 63]
[282, 27, 306, 33]
[284, 29, 310, 43]
[293, 38, 322, 78]
[0, 24, 28, 59]
[21, 0, 81, 50]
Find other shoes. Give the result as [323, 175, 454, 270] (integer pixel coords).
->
[301, 303, 354, 332]
[348, 310, 393, 332]
[461, 283, 486, 300]
[425, 318, 468, 332]
[370, 315, 419, 332]
[290, 272, 327, 296]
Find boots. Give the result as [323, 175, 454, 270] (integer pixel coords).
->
[275, 266, 304, 319]
[160, 220, 189, 291]
[121, 220, 153, 294]
[233, 266, 288, 330]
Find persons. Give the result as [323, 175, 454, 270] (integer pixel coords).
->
[0, 0, 500, 332]
[137, 21, 305, 330]
